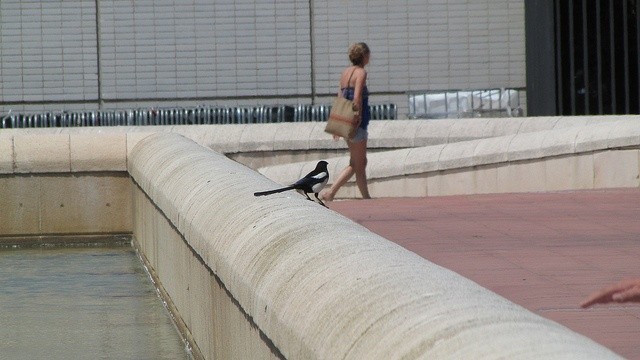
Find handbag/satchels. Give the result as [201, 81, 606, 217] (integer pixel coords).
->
[323, 65, 362, 140]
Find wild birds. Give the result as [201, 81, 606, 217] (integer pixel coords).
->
[254, 161, 329, 209]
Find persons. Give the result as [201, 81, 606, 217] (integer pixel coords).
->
[320, 43, 370, 200]
[581, 278, 640, 309]
